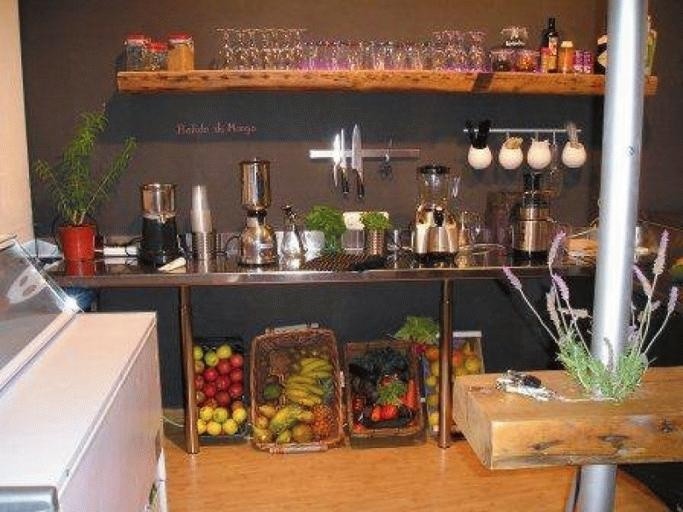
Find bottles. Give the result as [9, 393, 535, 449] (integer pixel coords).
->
[542, 18, 560, 72]
[123, 32, 195, 71]
[648, 15, 656, 75]
[558, 40, 575, 73]
[596, 9, 608, 74]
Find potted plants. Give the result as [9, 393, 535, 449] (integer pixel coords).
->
[34, 105, 135, 261]
[360, 211, 391, 250]
[302, 205, 345, 253]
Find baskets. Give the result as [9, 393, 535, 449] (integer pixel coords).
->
[342, 339, 425, 439]
[249, 322, 345, 454]
[193, 334, 249, 444]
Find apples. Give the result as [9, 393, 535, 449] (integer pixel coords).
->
[193, 344, 247, 436]
[425, 345, 481, 426]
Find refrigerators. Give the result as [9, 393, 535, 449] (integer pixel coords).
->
[0, 312, 168, 511]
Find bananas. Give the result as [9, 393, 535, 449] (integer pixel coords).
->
[297, 357, 334, 379]
[284, 376, 324, 407]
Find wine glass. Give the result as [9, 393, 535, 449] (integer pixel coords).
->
[213, 27, 491, 70]
[458, 210, 481, 265]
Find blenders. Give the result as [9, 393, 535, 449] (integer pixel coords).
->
[138, 182, 178, 266]
[411, 163, 459, 260]
[237, 160, 279, 265]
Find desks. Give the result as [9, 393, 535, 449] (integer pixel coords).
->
[48, 233, 594, 454]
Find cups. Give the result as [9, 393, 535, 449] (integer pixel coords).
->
[500, 27, 529, 48]
[516, 48, 541, 71]
[192, 230, 216, 261]
[191, 185, 213, 233]
[281, 224, 308, 255]
[491, 48, 515, 70]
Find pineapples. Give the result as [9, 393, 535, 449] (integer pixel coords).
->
[312, 379, 336, 441]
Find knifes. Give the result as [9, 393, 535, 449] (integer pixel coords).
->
[340, 129, 350, 194]
[352, 123, 364, 198]
